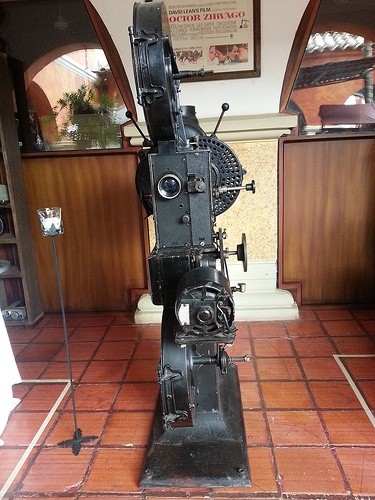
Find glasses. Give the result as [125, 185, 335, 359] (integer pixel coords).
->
[210, 51, 217, 55]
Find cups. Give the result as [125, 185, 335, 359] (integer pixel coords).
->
[37, 206, 64, 233]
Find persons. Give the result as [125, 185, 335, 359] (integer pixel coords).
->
[174, 49, 199, 65]
[209, 44, 248, 65]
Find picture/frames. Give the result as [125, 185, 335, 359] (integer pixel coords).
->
[145, 0, 262, 83]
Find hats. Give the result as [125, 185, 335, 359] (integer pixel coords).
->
[239, 43, 248, 49]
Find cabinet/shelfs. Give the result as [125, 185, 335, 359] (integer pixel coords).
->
[0, 53, 44, 328]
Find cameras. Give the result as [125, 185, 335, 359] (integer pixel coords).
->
[127, 0, 253, 486]
